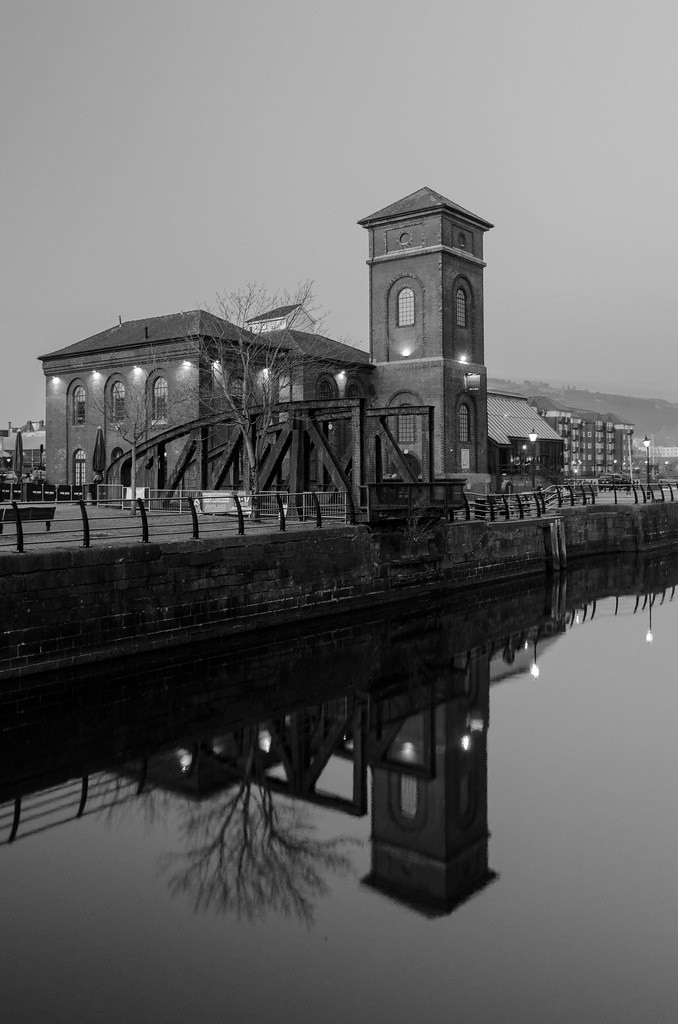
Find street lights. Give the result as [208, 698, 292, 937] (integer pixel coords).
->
[646, 593, 654, 643]
[528, 428, 539, 491]
[531, 643, 539, 679]
[642, 435, 651, 499]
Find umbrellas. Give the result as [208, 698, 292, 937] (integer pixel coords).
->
[91, 425, 107, 484]
[12, 428, 24, 485]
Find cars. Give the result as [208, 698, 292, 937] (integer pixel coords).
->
[599, 473, 625, 489]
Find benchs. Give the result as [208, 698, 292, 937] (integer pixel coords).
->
[0, 507, 56, 534]
